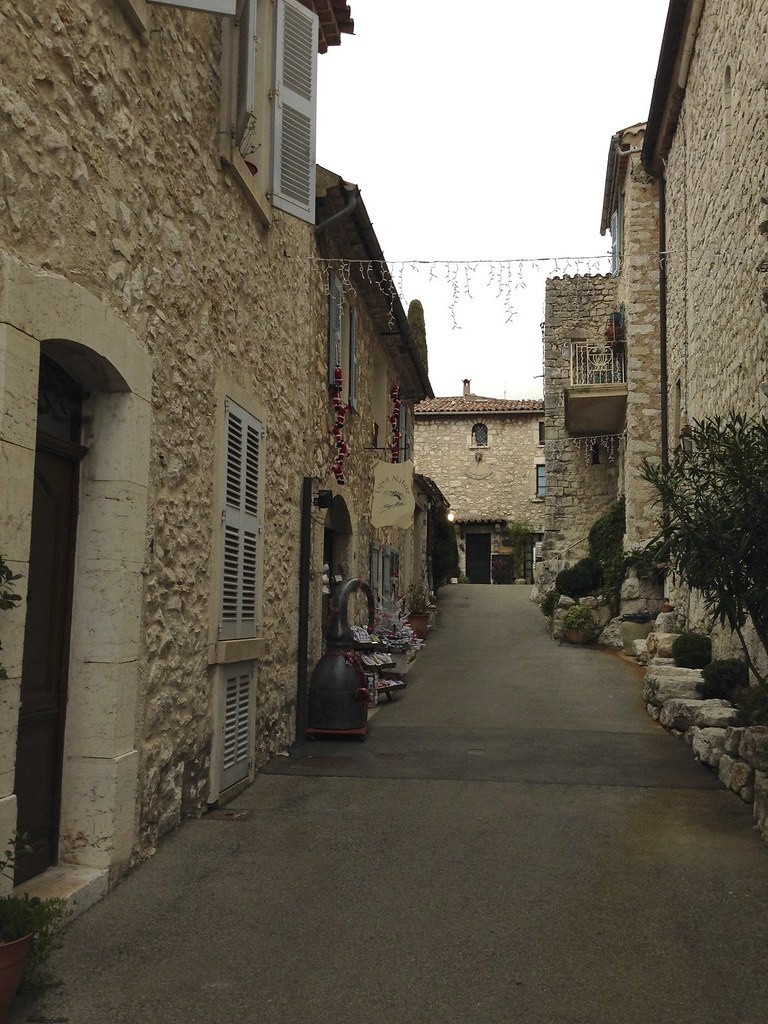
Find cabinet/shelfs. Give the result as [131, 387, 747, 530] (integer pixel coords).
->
[352, 638, 409, 703]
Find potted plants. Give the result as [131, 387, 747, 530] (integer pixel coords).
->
[558, 604, 595, 647]
[0, 892, 74, 1024]
[375, 584, 426, 675]
[404, 585, 437, 640]
